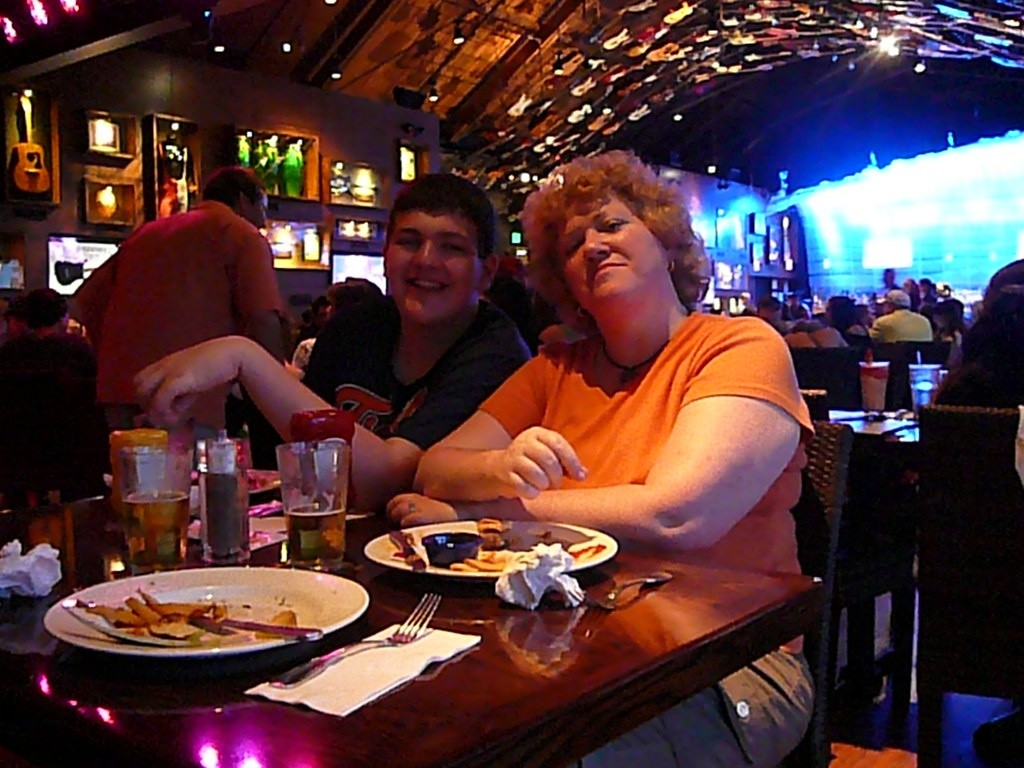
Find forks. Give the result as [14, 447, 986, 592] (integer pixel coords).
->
[568, 572, 673, 612]
[251, 593, 443, 688]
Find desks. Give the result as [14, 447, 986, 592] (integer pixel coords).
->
[0, 511, 824, 768]
[828, 409, 920, 733]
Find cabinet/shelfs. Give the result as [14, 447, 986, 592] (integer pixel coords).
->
[80, 110, 392, 270]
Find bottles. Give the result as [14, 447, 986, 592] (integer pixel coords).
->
[88, 112, 379, 265]
[290, 409, 355, 532]
[102, 430, 169, 580]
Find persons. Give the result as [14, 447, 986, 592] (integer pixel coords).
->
[388, 151, 815, 768]
[904, 258, 1024, 768]
[287, 276, 382, 374]
[718, 268, 984, 347]
[134, 172, 530, 510]
[68, 167, 286, 480]
[0, 288, 108, 513]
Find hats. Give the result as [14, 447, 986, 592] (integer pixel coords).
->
[885, 289, 912, 308]
[990, 260, 1024, 296]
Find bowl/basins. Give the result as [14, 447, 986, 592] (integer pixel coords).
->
[420, 533, 482, 568]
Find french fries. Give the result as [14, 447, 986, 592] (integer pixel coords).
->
[255, 610, 296, 638]
[449, 558, 504, 572]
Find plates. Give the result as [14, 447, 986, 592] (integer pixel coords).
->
[44, 568, 370, 656]
[248, 469, 281, 496]
[364, 521, 618, 578]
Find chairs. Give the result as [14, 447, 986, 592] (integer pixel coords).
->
[791, 341, 1024, 768]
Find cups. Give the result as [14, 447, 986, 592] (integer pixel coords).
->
[275, 441, 350, 574]
[195, 438, 251, 565]
[120, 445, 191, 575]
[858, 361, 889, 415]
[909, 363, 942, 421]
[65, 319, 83, 337]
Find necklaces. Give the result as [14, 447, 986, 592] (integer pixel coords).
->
[597, 339, 668, 383]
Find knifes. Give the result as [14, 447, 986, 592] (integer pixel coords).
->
[204, 611, 324, 641]
[389, 529, 426, 573]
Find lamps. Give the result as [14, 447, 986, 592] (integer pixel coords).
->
[329, 22, 343, 80]
[452, 13, 467, 44]
[428, 77, 439, 103]
[554, 52, 565, 76]
[281, 1, 311, 53]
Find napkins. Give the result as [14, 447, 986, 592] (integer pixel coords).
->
[187, 513, 368, 553]
[244, 622, 484, 719]
[494, 556, 585, 612]
[0, 539, 63, 601]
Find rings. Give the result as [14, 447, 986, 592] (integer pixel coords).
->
[409, 504, 415, 512]
